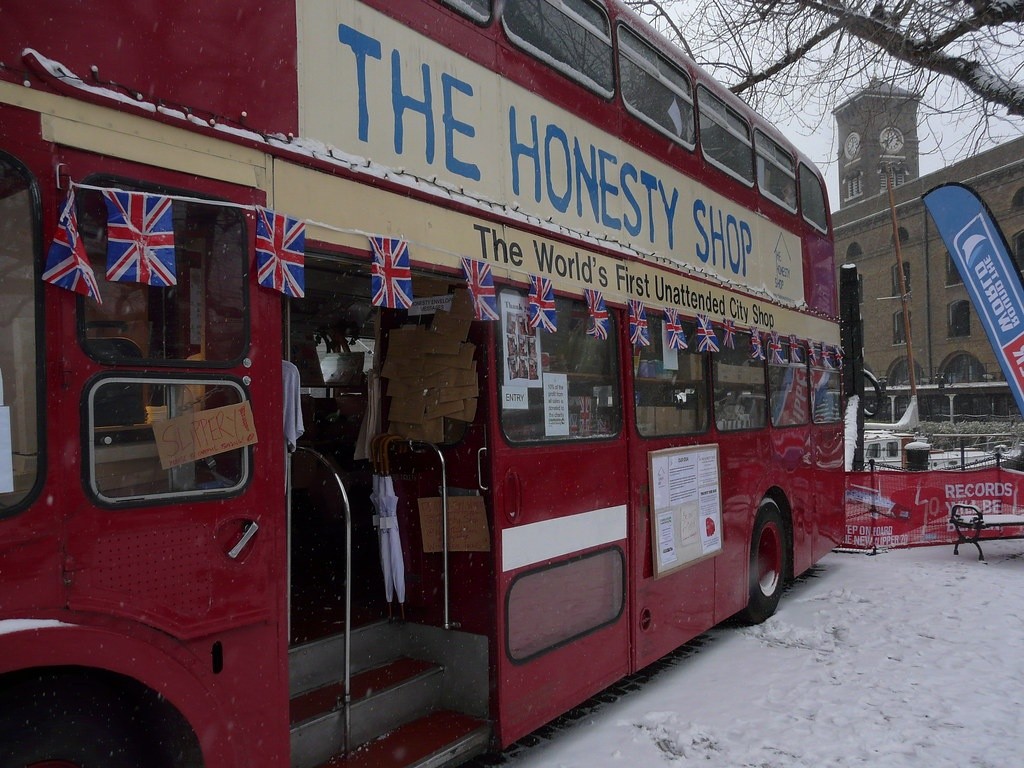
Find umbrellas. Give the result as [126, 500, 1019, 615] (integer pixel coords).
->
[369, 432, 409, 626]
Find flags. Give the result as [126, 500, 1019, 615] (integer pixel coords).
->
[569, 397, 612, 435]
[39, 181, 843, 370]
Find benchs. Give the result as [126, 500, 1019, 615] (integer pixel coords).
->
[950, 504, 1024, 561]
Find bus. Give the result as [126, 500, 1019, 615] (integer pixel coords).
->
[0, 0, 847, 768]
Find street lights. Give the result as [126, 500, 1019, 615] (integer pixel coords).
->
[876, 154, 920, 430]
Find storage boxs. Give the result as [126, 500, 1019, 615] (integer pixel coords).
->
[678, 352, 702, 380]
[713, 362, 764, 384]
[636, 405, 696, 436]
[93, 439, 169, 498]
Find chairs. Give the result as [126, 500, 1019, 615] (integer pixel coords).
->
[81, 335, 144, 441]
